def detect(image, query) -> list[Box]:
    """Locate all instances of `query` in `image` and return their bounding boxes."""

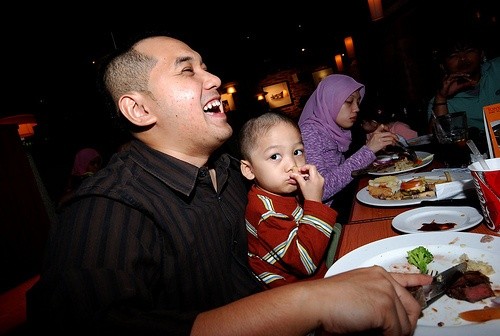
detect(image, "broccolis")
[408,247,434,273]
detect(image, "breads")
[369,176,400,200]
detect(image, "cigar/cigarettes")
[463,73,472,81]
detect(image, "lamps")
[367,0,384,21]
[335,53,344,71]
[344,34,356,60]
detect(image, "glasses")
[89,161,101,167]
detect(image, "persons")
[358,109,418,151]
[239,108,337,289]
[23,27,421,336]
[298,75,400,208]
[427,25,500,133]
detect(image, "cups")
[434,110,471,170]
[467,157,500,234]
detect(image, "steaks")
[445,272,492,303]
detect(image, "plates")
[367,151,434,176]
[405,129,456,145]
[355,172,471,206]
[323,231,500,336]
[391,205,484,233]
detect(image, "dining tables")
[314,159,500,336]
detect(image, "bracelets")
[433,102,447,106]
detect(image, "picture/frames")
[262,80,294,109]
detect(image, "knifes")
[408,262,467,309]
